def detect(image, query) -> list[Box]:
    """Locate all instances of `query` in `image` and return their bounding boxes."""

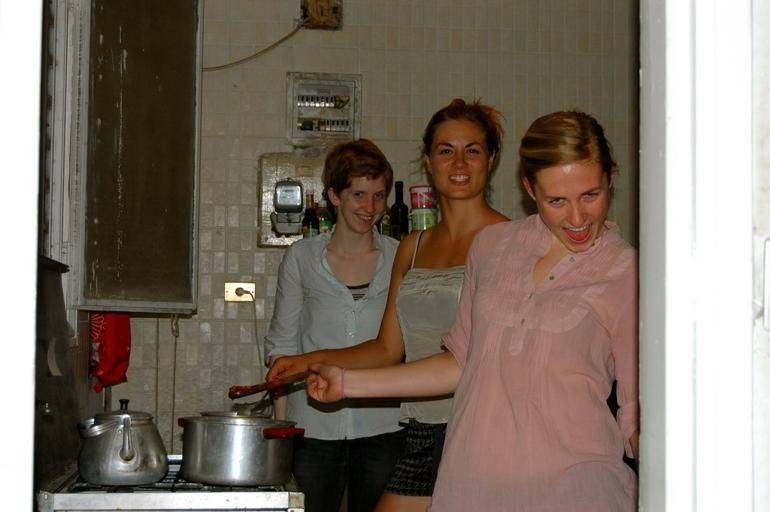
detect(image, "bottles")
[299,185,320,238]
[390,179,409,243]
[315,192,333,236]
[407,183,440,232]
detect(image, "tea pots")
[82,394,169,488]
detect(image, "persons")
[263,137,412,512]
[303,109,640,512]
[265,86,511,512]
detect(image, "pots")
[178,408,301,485]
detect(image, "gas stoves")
[51,449,305,509]
